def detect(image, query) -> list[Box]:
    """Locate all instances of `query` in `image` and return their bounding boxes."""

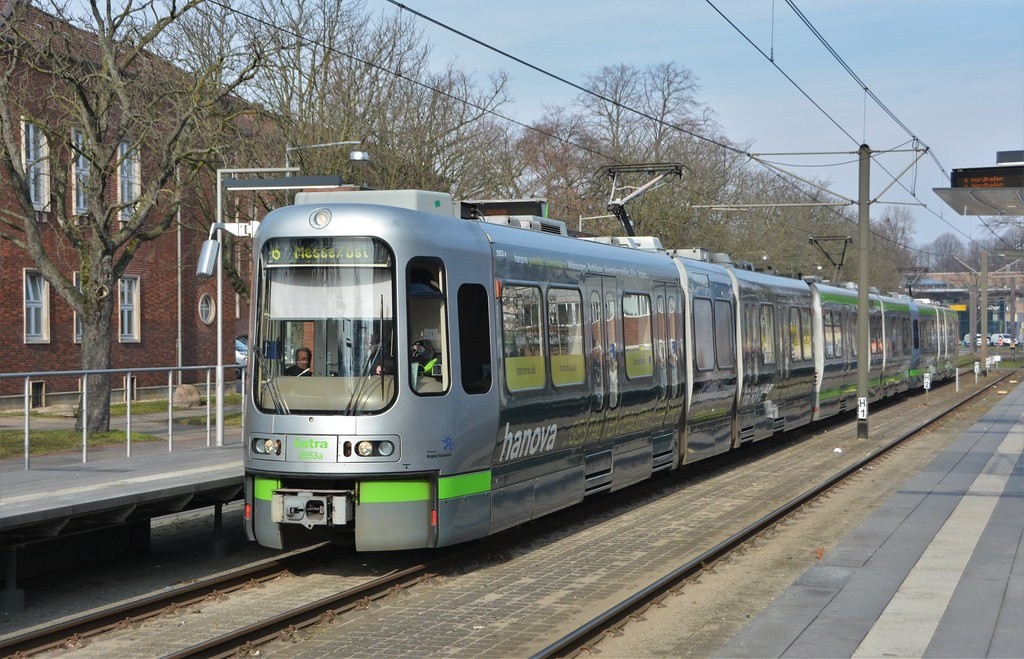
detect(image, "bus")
[194,161,962,553]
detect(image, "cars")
[234,335,249,380]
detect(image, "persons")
[341,321,394,374]
[284,347,312,377]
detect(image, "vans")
[963,333,991,348]
[990,333,1019,346]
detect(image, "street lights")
[285,139,369,207]
